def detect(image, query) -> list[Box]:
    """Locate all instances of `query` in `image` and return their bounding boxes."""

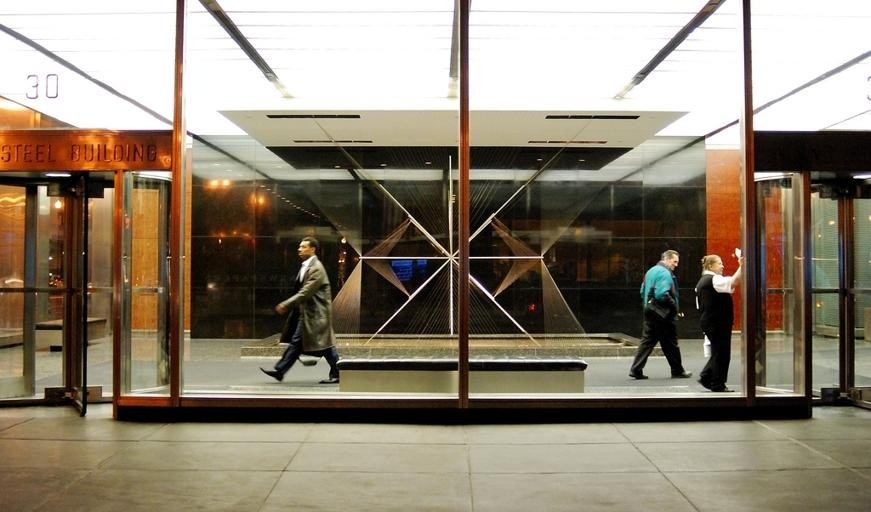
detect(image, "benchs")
[336,356,588,395]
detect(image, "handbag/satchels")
[644,297,674,328]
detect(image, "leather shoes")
[318,377,339,384]
[671,371,692,378]
[258,366,284,382]
[629,370,648,379]
[697,377,735,392]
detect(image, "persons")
[628,250,692,380]
[695,255,744,392]
[259,237,340,384]
